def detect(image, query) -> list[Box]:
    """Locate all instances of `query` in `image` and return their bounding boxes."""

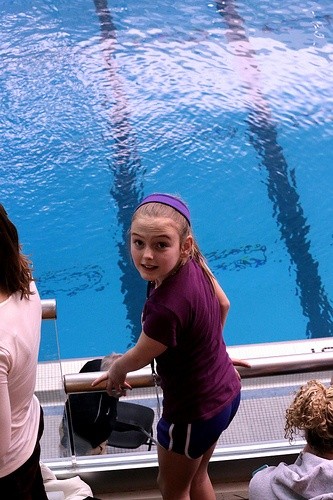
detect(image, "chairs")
[107,401,167,452]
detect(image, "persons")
[0,204,48,500]
[248,380,333,500]
[91,192,241,500]
